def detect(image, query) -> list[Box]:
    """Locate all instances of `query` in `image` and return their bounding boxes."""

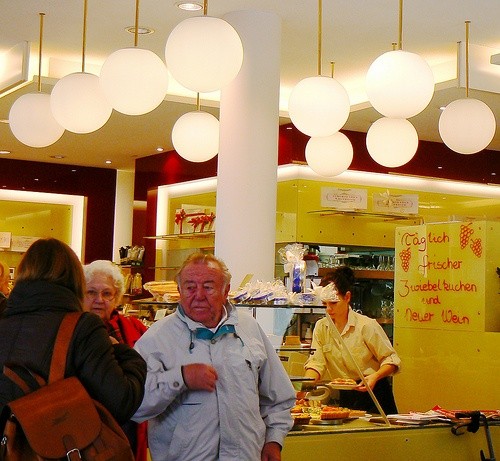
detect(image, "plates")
[326,383,360,392]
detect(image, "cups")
[274,297,287,305]
[302,293,316,303]
[286,336,300,346]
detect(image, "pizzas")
[290,406,311,413]
[331,378,357,385]
[320,405,351,420]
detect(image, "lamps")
[8,0,496,177]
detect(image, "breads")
[294,391,318,407]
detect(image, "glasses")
[83,287,117,302]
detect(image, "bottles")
[305,321,315,345]
[134,272,143,294]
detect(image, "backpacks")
[0,311,135,461]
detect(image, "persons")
[129,254,297,461]
[0,238,148,461]
[81,260,149,461]
[305,266,402,415]
[0,262,10,304]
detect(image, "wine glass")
[378,297,394,318]
[366,254,395,270]
